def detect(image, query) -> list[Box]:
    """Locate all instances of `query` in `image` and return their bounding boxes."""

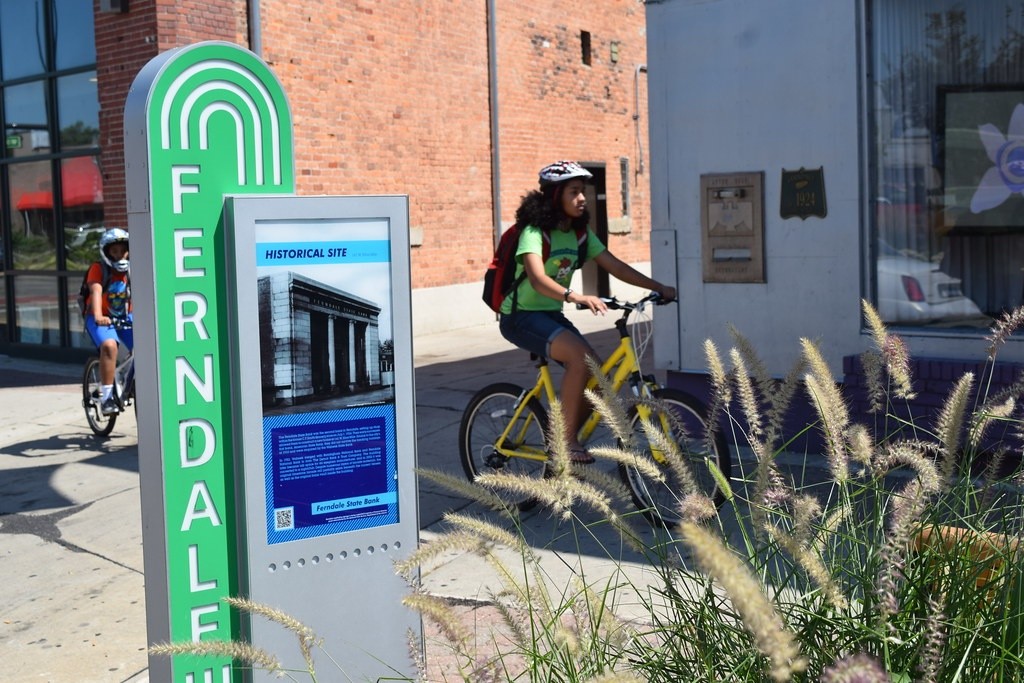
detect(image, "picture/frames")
[933,85,1024,235]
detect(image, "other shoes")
[101,397,120,414]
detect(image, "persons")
[497,160,677,463]
[78,228,134,414]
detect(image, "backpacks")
[484,219,589,314]
[77,261,131,319]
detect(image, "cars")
[62,214,105,250]
[872,182,980,328]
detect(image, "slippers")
[545,446,596,465]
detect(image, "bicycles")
[81,317,137,437]
[456,288,732,533]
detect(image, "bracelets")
[563,289,574,304]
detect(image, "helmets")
[99,228,129,272]
[539,161,594,190]
